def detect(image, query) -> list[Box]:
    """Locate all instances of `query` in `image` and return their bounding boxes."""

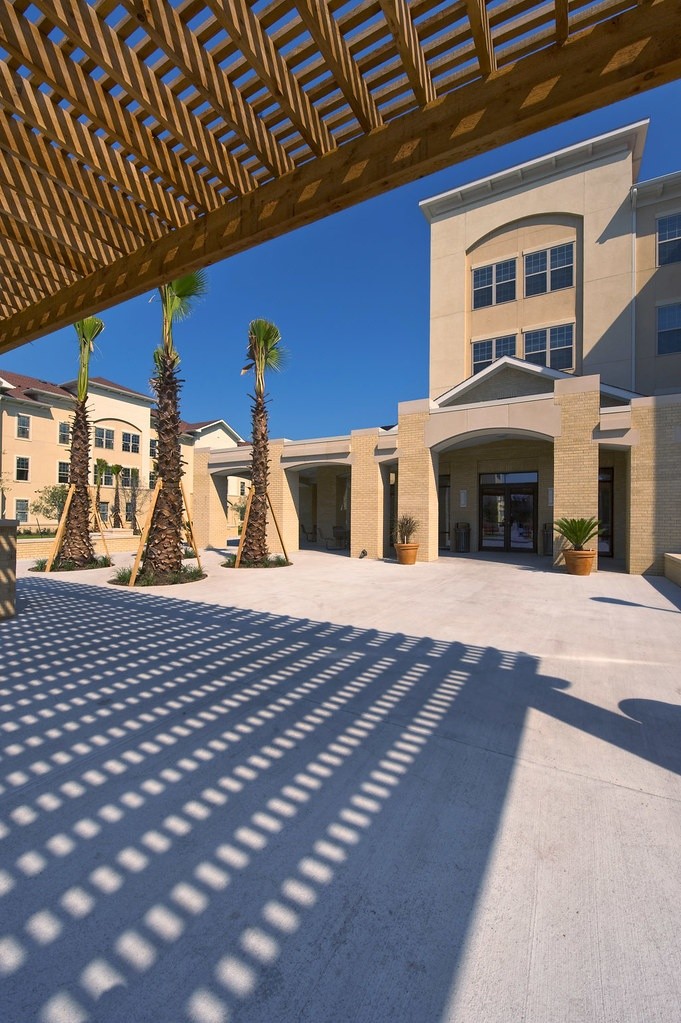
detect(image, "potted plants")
[390,515,422,566]
[550,515,610,575]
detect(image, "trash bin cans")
[453,521,471,553]
[543,523,554,556]
